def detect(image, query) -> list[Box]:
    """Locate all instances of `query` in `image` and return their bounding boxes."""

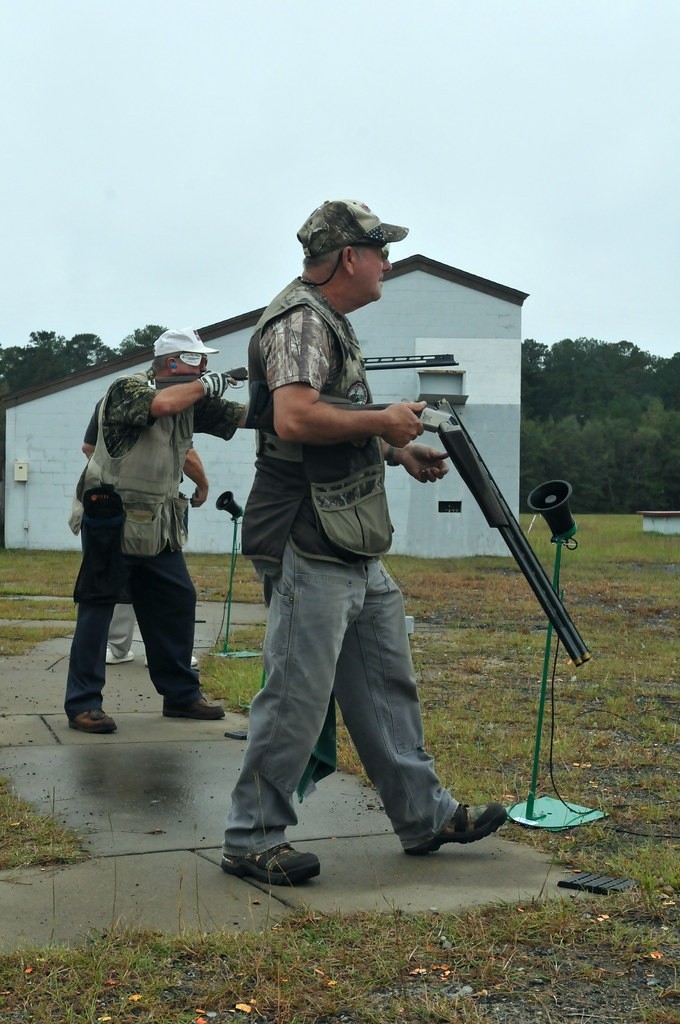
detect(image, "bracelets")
[387,446,400,466]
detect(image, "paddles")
[143,350,460,390]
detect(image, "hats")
[297,200,409,257]
[153,327,220,357]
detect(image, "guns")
[315,393,592,668]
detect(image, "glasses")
[174,352,207,367]
[349,242,390,261]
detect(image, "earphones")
[170,362,177,369]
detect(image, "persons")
[63,327,248,734]
[220,199,508,886]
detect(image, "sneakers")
[163,697,225,719]
[69,708,117,733]
[404,803,507,855]
[222,842,320,885]
